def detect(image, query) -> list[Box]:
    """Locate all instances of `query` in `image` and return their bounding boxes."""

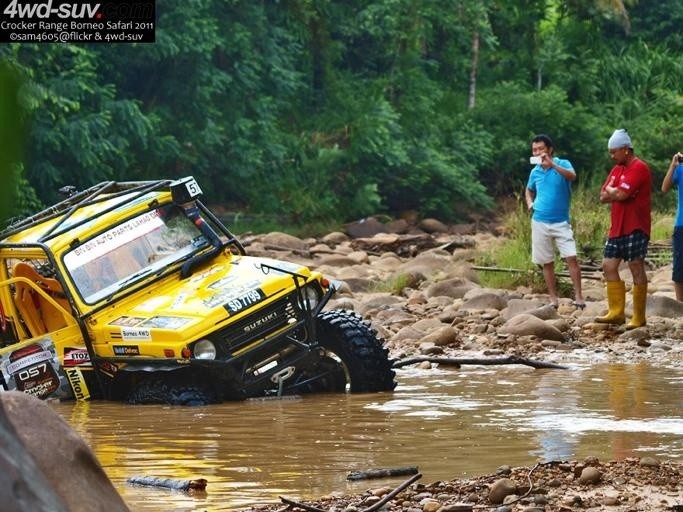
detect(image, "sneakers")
[570,300,586,308]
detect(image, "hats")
[608,129,633,150]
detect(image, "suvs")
[0,175,396,407]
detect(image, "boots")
[594,280,626,323]
[625,283,647,330]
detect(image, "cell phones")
[529,158,543,165]
[678,157,683,163]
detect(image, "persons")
[535,364,658,462]
[662,152,683,303]
[525,135,586,307]
[595,129,655,329]
